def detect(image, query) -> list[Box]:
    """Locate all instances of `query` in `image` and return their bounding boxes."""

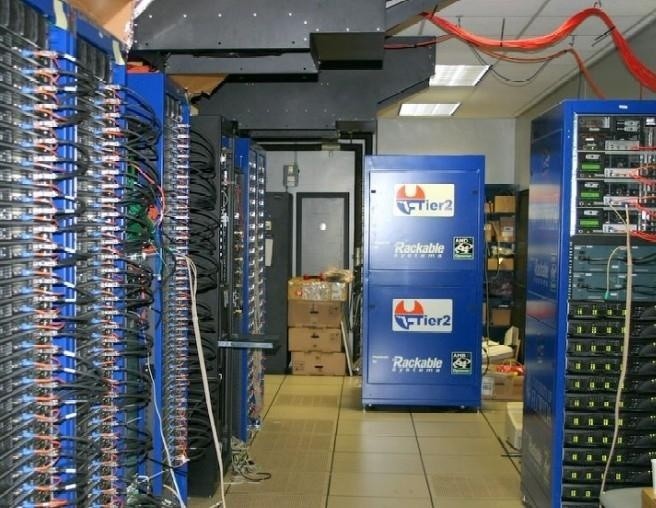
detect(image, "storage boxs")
[287,277,349,377]
[482,196,525,401]
